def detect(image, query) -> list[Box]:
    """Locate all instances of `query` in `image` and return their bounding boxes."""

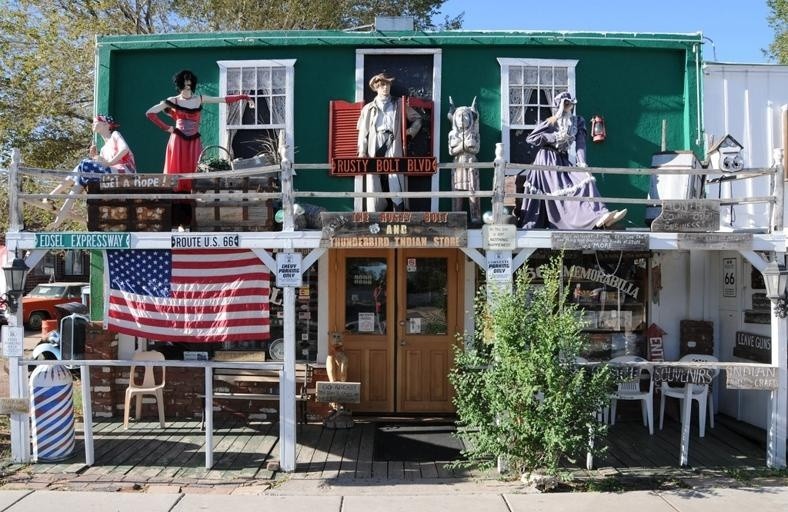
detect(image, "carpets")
[371,426,468,461]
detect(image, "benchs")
[199,364,313,427]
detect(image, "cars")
[1,281,89,333]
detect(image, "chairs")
[123,351,166,428]
[535,353,720,468]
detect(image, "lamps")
[590,115,607,143]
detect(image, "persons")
[446,106,482,223]
[518,91,628,230]
[40,113,136,228]
[354,72,422,212]
[144,70,251,207]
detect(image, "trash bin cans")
[29,364,75,463]
[55,302,88,333]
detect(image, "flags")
[101,249,271,343]
